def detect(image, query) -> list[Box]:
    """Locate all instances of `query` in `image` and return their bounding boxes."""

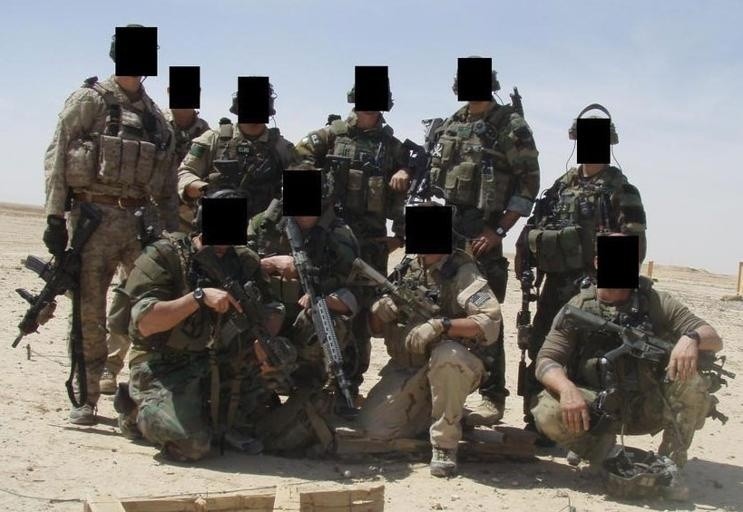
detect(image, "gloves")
[404,321,441,356]
[43,215,68,260]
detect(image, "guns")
[517,232,543,432]
[282,216,357,420]
[346,258,494,367]
[11,203,102,349]
[193,277,298,391]
[556,304,737,378]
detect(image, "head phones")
[453,70,500,96]
[569,104,619,145]
[280,169,333,199]
[230,90,276,116]
[347,85,394,110]
[109,24,145,63]
[193,188,240,232]
[403,184,456,215]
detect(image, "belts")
[73,192,147,210]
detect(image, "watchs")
[193,286,206,308]
[441,316,451,335]
[491,223,507,238]
[683,329,700,345]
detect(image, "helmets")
[600,456,659,497]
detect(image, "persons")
[41,27,178,423]
[404,54,540,420]
[513,115,648,447]
[293,71,427,412]
[175,80,301,222]
[354,200,502,479]
[114,188,286,460]
[161,80,213,233]
[527,233,724,502]
[246,162,361,414]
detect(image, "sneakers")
[429,450,460,478]
[465,394,504,426]
[71,399,95,425]
[225,428,261,453]
[99,366,117,393]
[118,407,141,438]
